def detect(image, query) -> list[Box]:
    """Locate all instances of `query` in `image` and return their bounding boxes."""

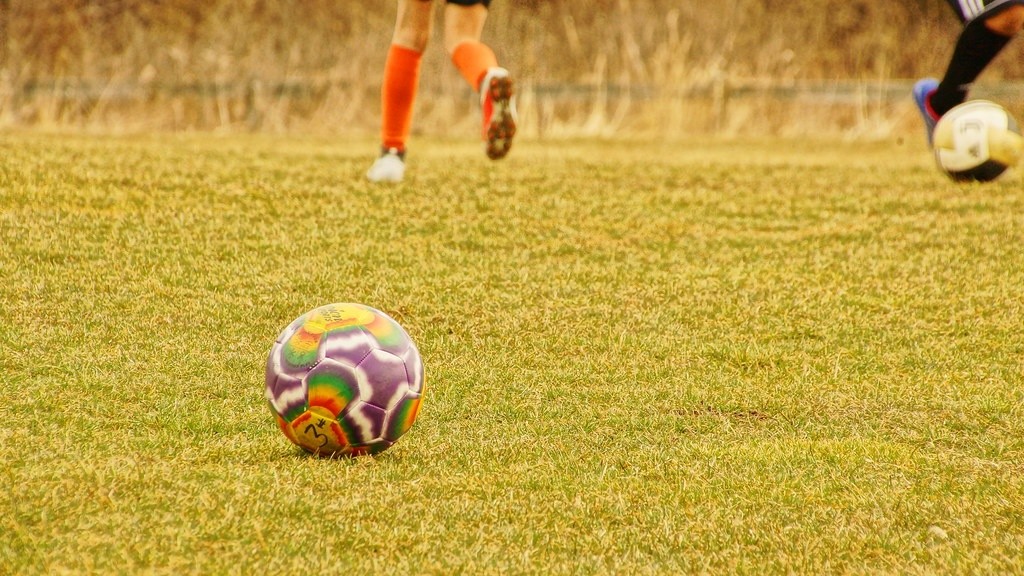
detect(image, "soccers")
[932,99,1023,183]
[264,302,427,457]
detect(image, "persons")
[914,0,1024,149]
[365,1,517,185]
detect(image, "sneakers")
[367,147,405,183]
[913,78,941,157]
[479,67,518,160]
